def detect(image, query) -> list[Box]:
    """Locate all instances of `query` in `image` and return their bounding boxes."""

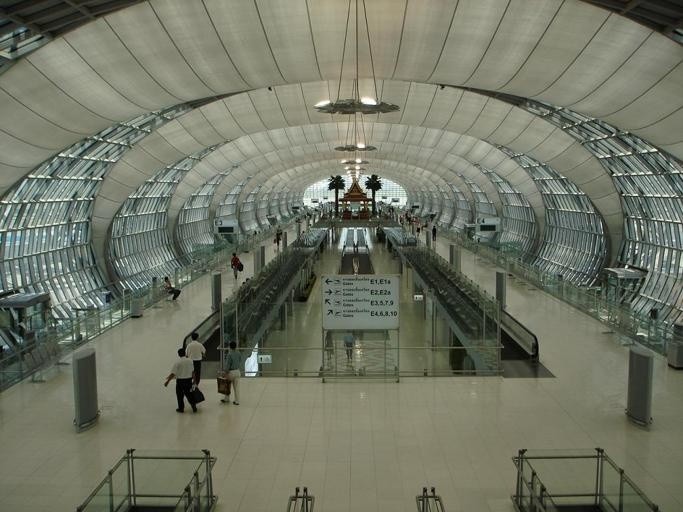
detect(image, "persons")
[162,348,197,412]
[274,229,281,246]
[277,225,283,237]
[230,252,239,280]
[163,277,180,301]
[218,340,241,405]
[380,201,428,237]
[431,225,436,241]
[183,332,206,388]
[323,331,334,366]
[342,332,355,359]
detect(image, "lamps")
[313,1,399,153]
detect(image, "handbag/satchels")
[189,381,205,404]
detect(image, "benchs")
[23,337,70,383]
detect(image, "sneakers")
[176,408,185,413]
[192,407,198,413]
[220,399,231,404]
[233,401,240,405]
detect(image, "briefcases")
[216,374,231,395]
